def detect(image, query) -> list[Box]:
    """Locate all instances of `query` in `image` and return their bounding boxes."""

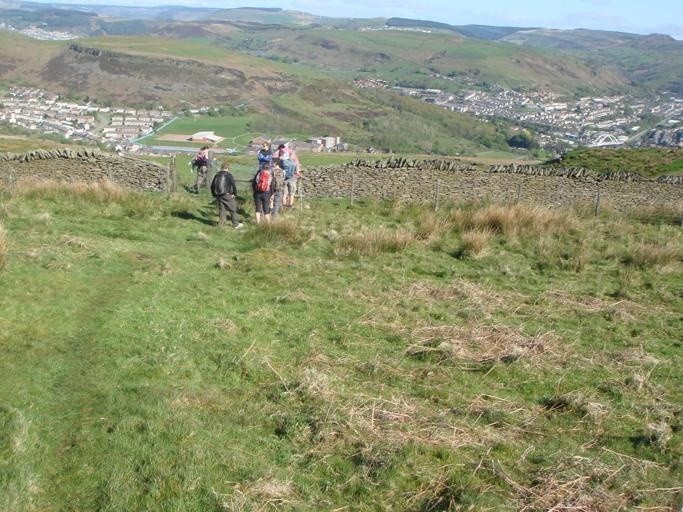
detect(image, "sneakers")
[233,223,243,229]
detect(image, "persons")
[251,142,301,226]
[194,145,211,194]
[210,161,243,229]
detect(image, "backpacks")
[272,169,284,192]
[255,169,270,193]
[214,172,229,196]
[282,159,296,176]
